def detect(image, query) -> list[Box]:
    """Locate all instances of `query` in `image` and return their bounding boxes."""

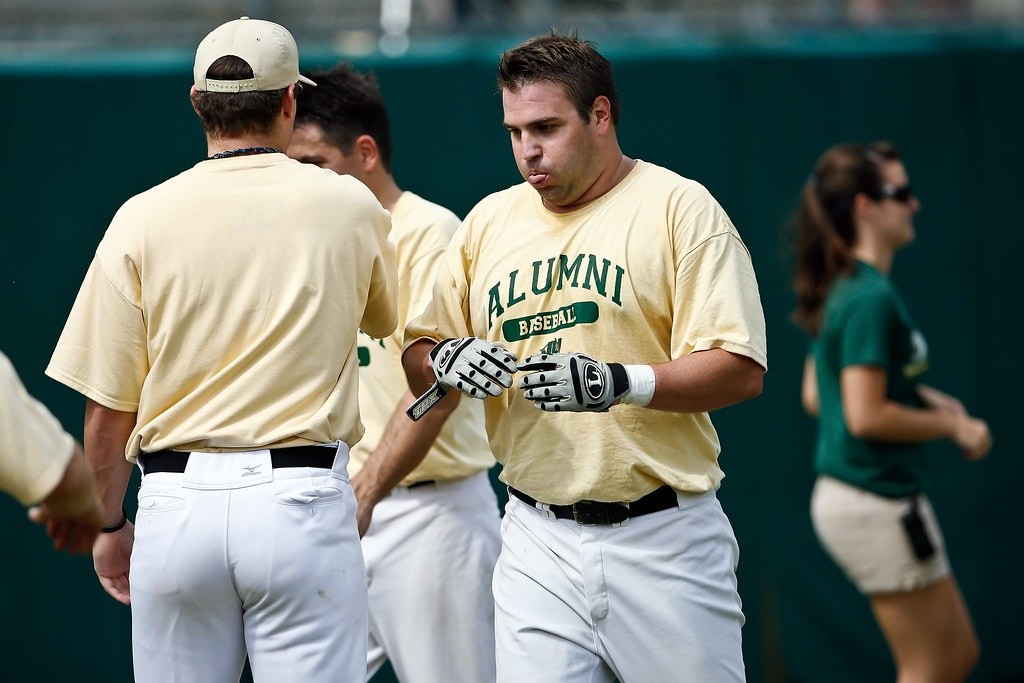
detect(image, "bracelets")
[101,507,128,533]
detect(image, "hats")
[192,17,318,94]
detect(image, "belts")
[405,480,437,491]
[135,445,338,475]
[508,484,678,527]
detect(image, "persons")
[400,33,766,683]
[801,140,989,683]
[0,13,501,683]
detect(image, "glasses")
[877,184,913,204]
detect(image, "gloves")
[403,337,519,422]
[515,351,633,414]
